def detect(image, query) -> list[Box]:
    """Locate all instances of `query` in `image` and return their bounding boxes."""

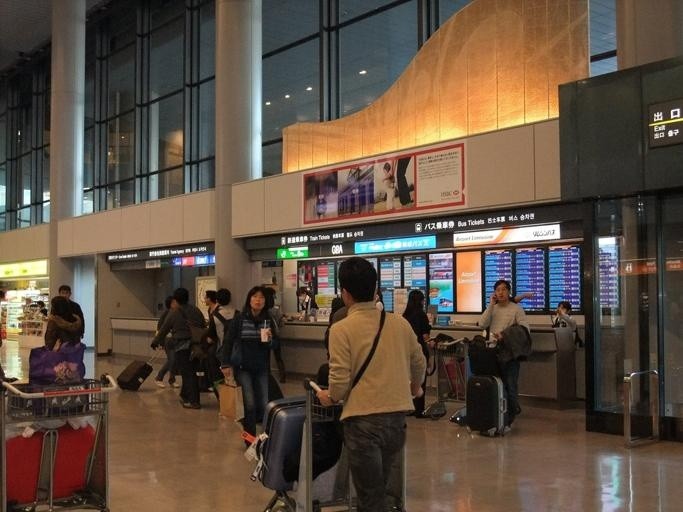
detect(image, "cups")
[259,328,270,343]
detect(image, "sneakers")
[179,399,201,409]
[155,380,166,388]
[170,382,180,387]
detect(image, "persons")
[202,288,219,341]
[401,289,436,420]
[34,300,48,317]
[43,294,81,351]
[374,289,383,302]
[315,257,427,512]
[381,157,413,209]
[265,286,282,329]
[204,288,240,424]
[508,291,535,303]
[220,284,288,449]
[23,296,33,308]
[295,286,319,321]
[380,162,395,212]
[58,285,85,340]
[477,279,532,436]
[154,295,180,388]
[375,299,386,311]
[150,286,208,409]
[551,300,578,331]
[324,296,344,363]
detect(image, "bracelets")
[327,394,339,404]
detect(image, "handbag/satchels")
[422,402,447,421]
[215,340,243,366]
[217,370,245,421]
[434,333,456,354]
[29,344,87,416]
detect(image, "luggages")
[117,348,162,393]
[256,395,344,491]
[466,375,507,437]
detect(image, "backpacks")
[214,309,241,336]
[468,335,500,377]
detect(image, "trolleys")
[256,378,407,512]
[0,370,118,512]
[421,335,471,421]
